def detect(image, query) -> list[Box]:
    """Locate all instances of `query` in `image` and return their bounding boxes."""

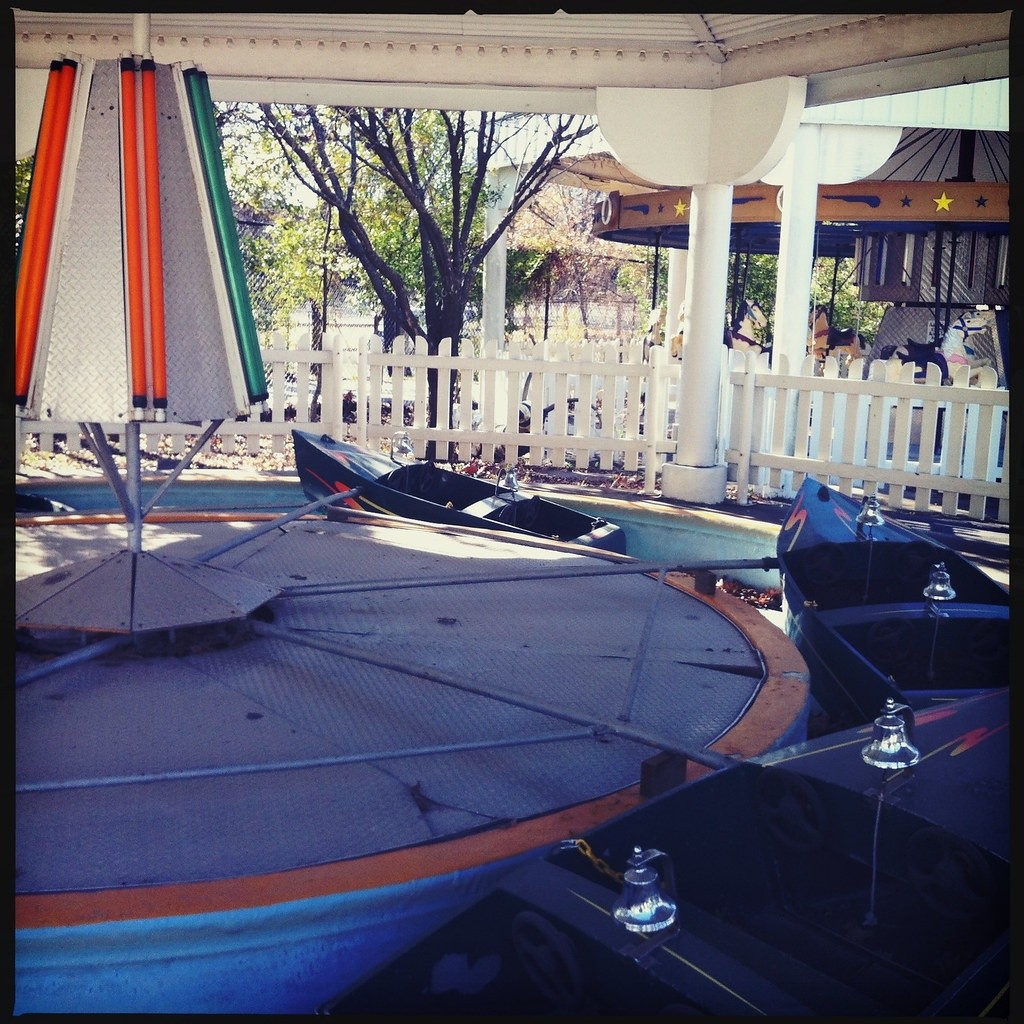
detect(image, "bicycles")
[501,395,626,474]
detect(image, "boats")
[285,419,625,564]
[777,466,1011,717]
[321,692,1024,1024]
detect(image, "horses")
[644,296,996,386]
